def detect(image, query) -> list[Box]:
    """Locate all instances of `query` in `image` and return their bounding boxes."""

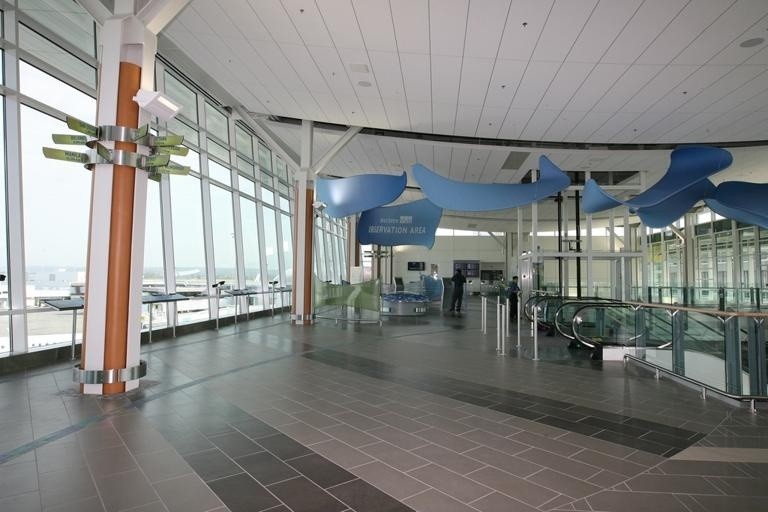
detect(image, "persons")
[507,276,520,323]
[449,268,466,312]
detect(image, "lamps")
[132,87,182,123]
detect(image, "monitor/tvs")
[407,262,425,271]
